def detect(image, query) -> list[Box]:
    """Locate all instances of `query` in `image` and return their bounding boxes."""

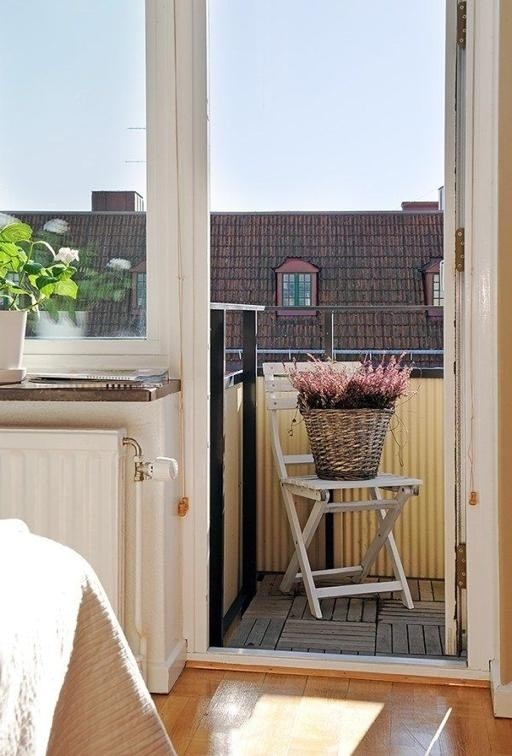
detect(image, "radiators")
[0,425,127,634]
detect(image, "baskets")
[296,393,396,482]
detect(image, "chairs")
[263,362,424,619]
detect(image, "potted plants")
[0,221,80,383]
[285,353,423,481]
[31,219,129,337]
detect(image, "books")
[27,367,170,384]
